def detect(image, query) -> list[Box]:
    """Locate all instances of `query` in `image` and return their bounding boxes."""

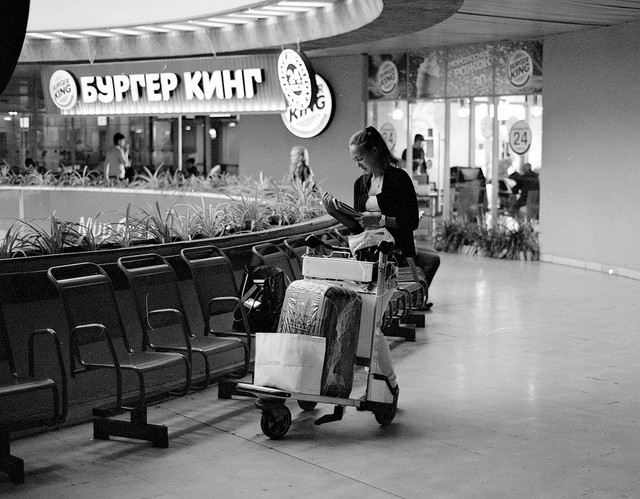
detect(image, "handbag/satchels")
[347,228,395,259]
[355,241,394,284]
[254,333,326,395]
[232,264,287,331]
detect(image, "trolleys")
[219,234,402,438]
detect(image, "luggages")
[277,279,362,398]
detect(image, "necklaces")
[373,176,381,188]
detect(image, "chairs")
[283,236,305,279]
[311,225,428,342]
[252,243,297,281]
[117,252,250,391]
[180,244,256,398]
[48,262,192,448]
[1,309,68,484]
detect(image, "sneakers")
[356,384,399,411]
[425,303,433,309]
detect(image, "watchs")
[379,215,386,228]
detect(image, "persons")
[401,134,428,174]
[207,164,226,180]
[321,126,421,405]
[513,163,540,210]
[185,158,197,177]
[288,145,315,192]
[104,132,130,180]
